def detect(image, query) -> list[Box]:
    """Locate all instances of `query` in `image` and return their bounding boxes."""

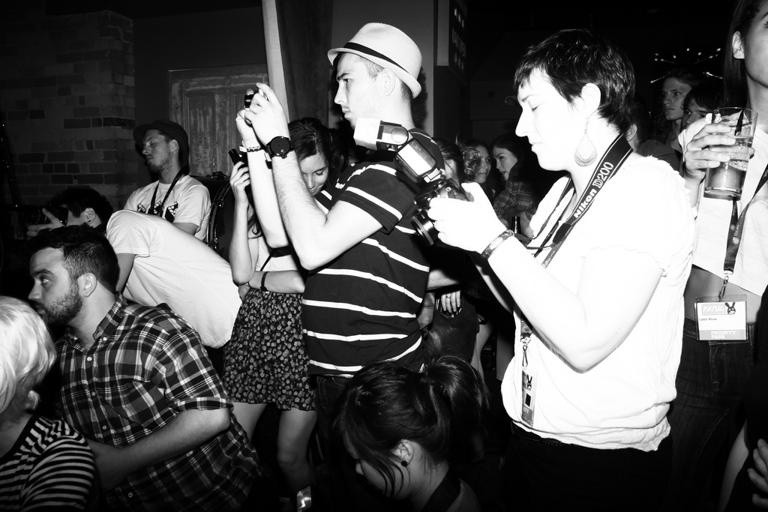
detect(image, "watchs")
[267,136,295,158]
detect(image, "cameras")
[240,88,261,112]
[2,199,66,242]
[276,479,314,511]
[376,119,472,248]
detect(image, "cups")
[703,105,758,201]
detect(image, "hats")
[133,120,188,151]
[327,22,422,99]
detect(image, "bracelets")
[241,141,263,152]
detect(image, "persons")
[415,0,767,511]
[224,117,334,511]
[27,228,285,511]
[235,21,446,512]
[332,355,490,512]
[0,295,96,512]
[26,188,246,380]
[122,121,211,243]
